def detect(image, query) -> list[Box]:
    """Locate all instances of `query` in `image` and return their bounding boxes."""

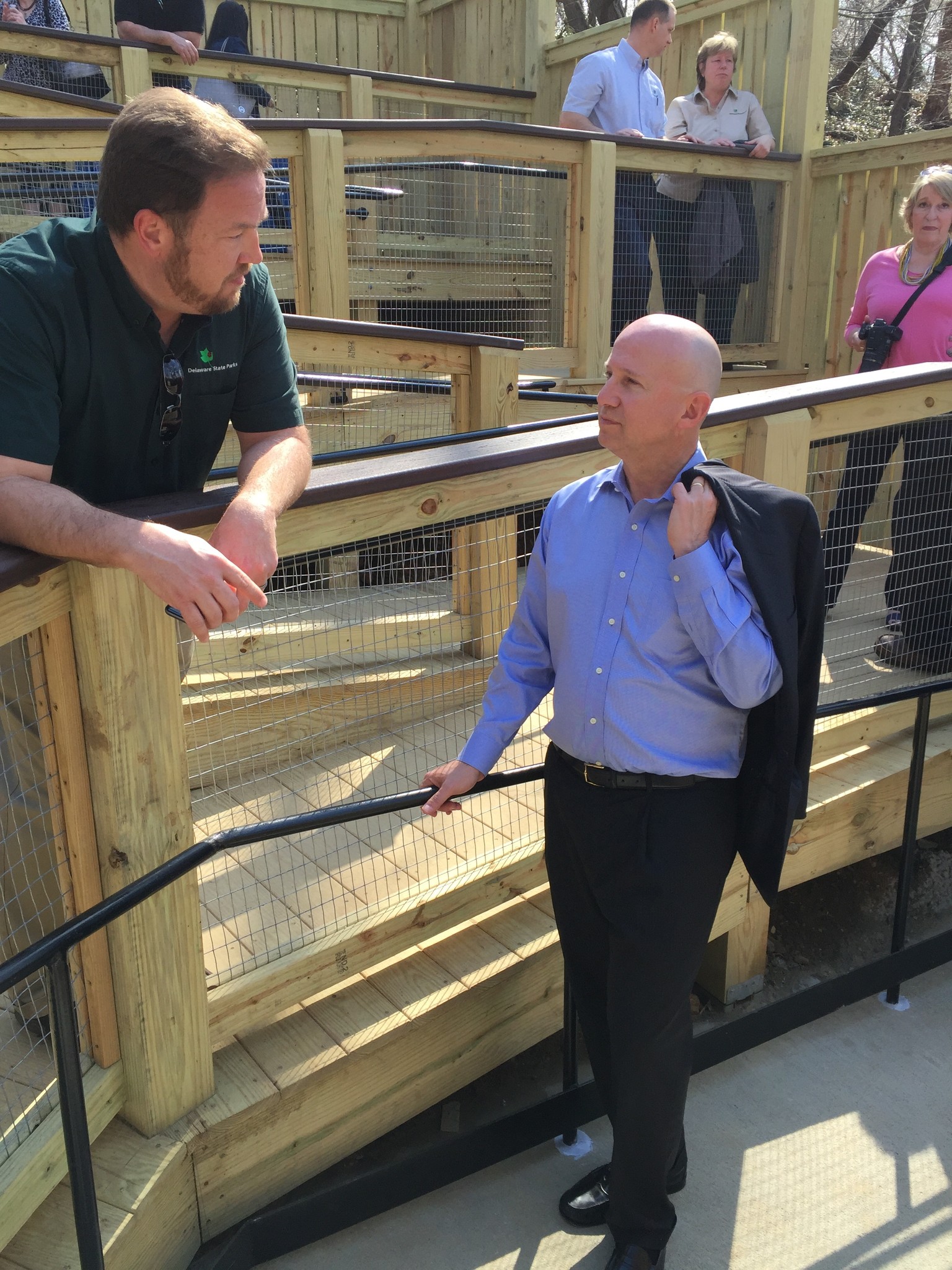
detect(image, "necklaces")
[17,0,36,11]
[899,233,952,286]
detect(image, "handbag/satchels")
[39,0,111,100]
[196,38,256,118]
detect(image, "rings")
[692,482,707,492]
[13,16,15,20]
[859,346,862,351]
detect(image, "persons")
[558,0,707,346]
[874,425,952,676]
[656,31,776,371]
[421,314,785,1270]
[0,86,315,1040]
[204,1,276,117]
[114,0,207,98]
[822,168,952,630]
[0,0,72,217]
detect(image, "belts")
[553,745,705,788]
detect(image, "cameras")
[858,318,903,373]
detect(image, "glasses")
[240,4,245,11]
[158,349,185,445]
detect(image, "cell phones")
[165,591,215,625]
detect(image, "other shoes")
[875,635,952,671]
[722,363,734,371]
[12,1010,52,1041]
[886,612,902,631]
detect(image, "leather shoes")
[605,1242,666,1270]
[558,1161,687,1227]
